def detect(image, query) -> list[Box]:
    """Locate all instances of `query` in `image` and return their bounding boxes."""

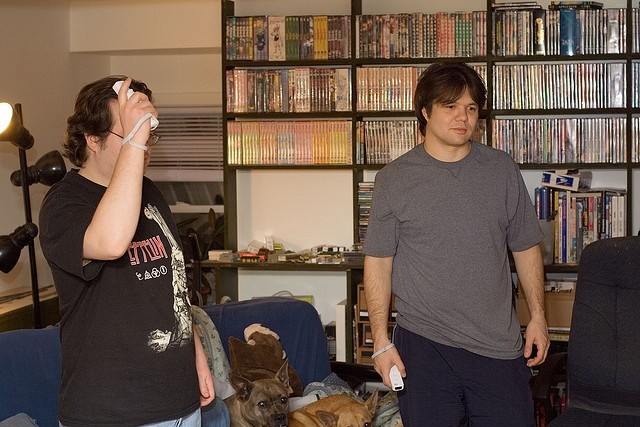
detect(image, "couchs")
[0,297,331,427]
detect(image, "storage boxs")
[513,278,578,328]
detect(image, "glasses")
[106,131,161,149]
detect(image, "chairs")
[545,237,639,427]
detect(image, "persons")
[38,74,215,426]
[363,59,550,426]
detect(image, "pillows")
[322,371,354,392]
[303,382,364,405]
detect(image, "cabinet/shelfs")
[218,0,638,346]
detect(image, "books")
[492,114,627,164]
[354,11,487,59]
[489,1,627,56]
[358,181,375,243]
[356,62,426,111]
[226,15,351,60]
[631,117,639,163]
[491,59,627,109]
[468,118,487,146]
[356,116,425,164]
[534,170,627,265]
[468,63,487,110]
[226,118,353,166]
[632,60,639,109]
[632,7,640,54]
[226,66,352,112]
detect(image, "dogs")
[288,386,379,427]
[222,358,294,427]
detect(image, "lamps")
[0,101,67,324]
